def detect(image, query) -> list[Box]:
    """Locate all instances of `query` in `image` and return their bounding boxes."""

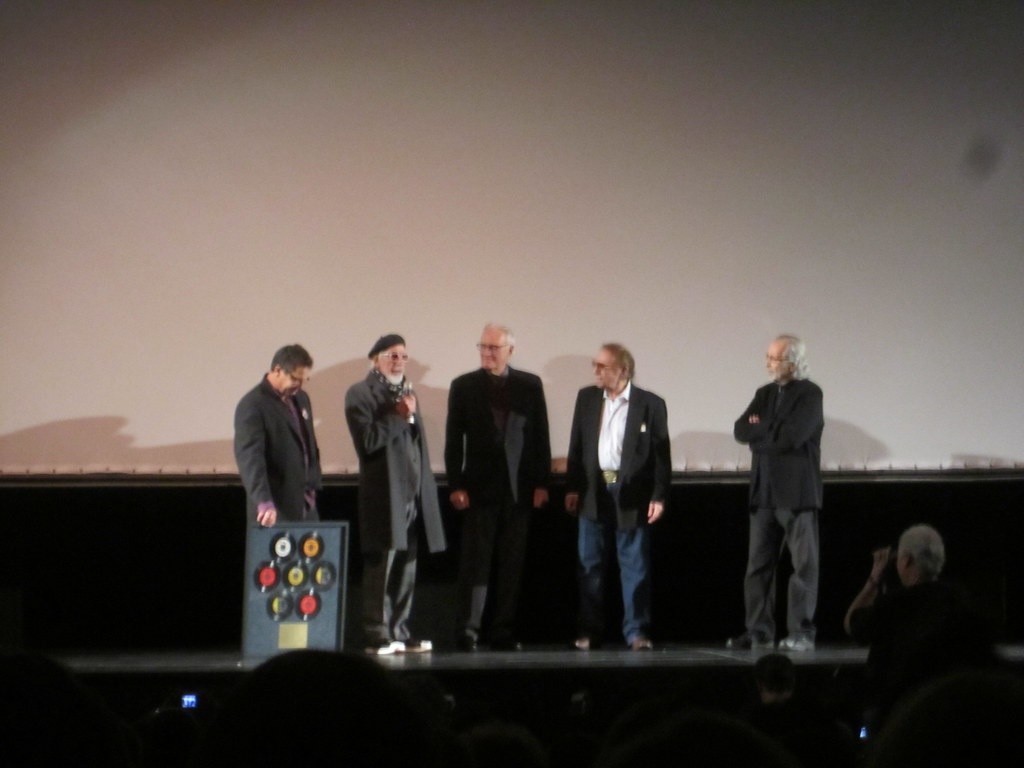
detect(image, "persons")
[443,322,552,653]
[0,661,1024,768]
[844,525,991,733]
[565,345,672,651]
[727,335,824,652]
[343,335,445,654]
[234,344,321,528]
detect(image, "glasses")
[376,353,409,362]
[476,343,510,352]
[281,366,309,384]
[592,359,624,370]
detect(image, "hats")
[368,335,405,358]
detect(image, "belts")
[599,470,620,483]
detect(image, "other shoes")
[457,640,478,652]
[491,638,522,652]
[625,635,652,651]
[367,642,409,655]
[725,633,774,651]
[406,637,432,655]
[569,634,601,651]
[779,632,814,653]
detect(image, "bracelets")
[869,576,881,589]
